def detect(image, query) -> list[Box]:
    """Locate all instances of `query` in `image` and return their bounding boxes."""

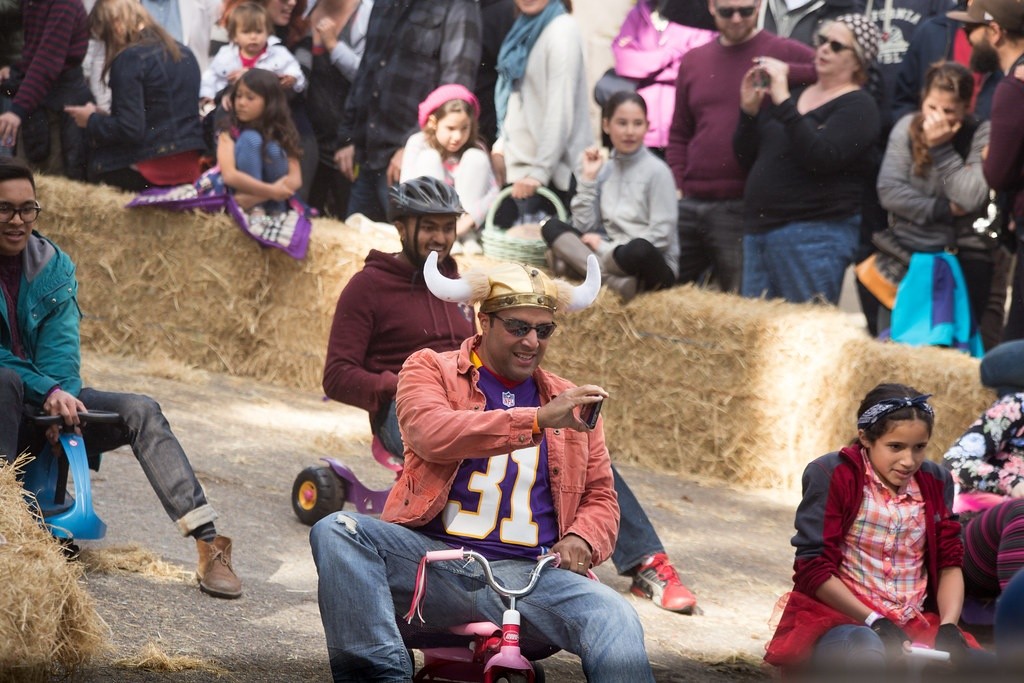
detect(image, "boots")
[545,242,626,279]
[551,231,636,304]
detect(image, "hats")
[945,0,1024,34]
[418,84,479,127]
[838,14,879,63]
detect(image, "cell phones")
[579,394,604,430]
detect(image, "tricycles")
[403,549,559,683]
[291,432,408,525]
[13,406,123,561]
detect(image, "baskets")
[479,186,568,266]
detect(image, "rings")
[577,562,584,566]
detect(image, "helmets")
[422,251,603,314]
[387,176,466,219]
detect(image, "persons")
[759,380,1001,682]
[0,1,1024,355]
[306,245,667,683]
[323,174,699,616]
[0,161,247,600]
[939,390,1024,615]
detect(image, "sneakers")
[629,551,695,613]
[194,536,242,598]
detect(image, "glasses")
[960,22,986,38]
[0,201,41,224]
[488,312,558,340]
[818,34,853,54]
[714,2,756,17]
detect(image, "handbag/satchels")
[856,252,908,310]
[593,72,640,107]
